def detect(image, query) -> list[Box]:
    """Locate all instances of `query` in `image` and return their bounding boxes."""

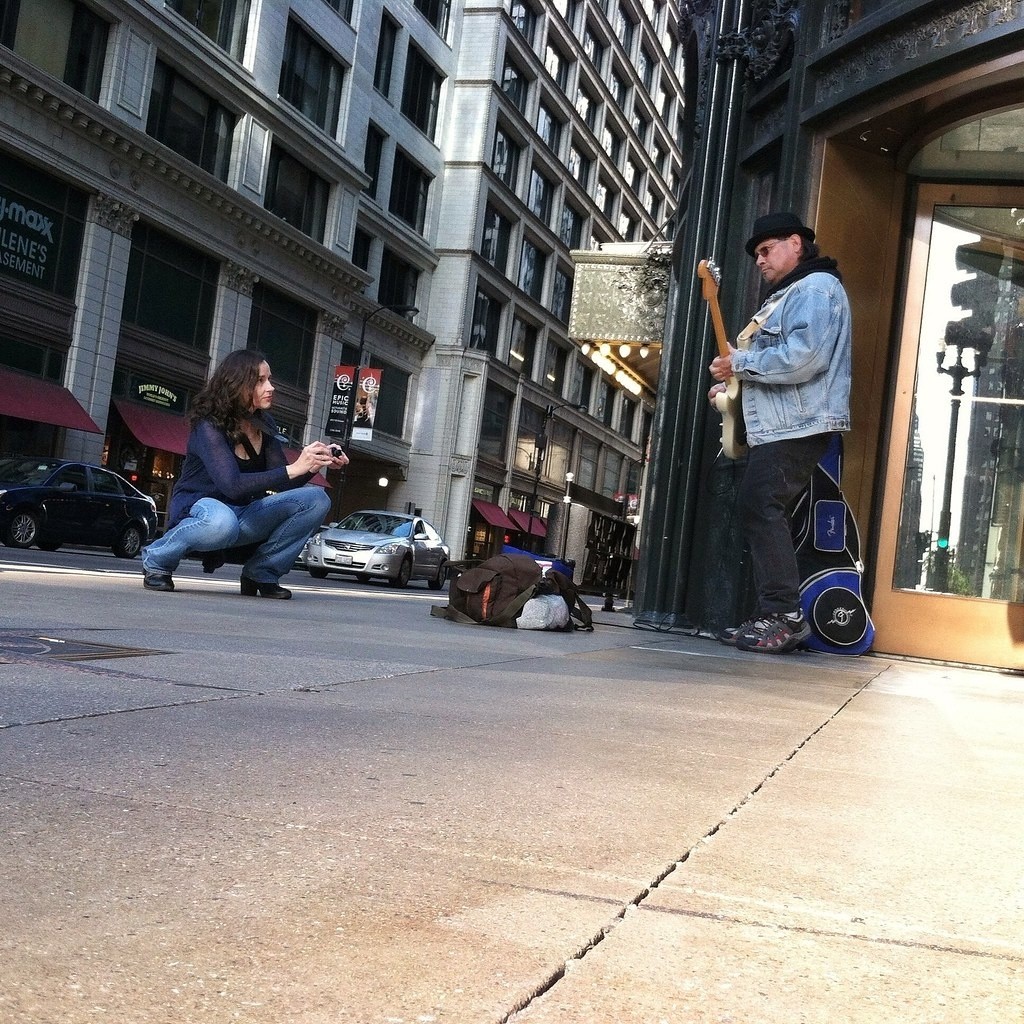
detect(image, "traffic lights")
[945,240,1004,347]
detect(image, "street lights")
[334,302,419,528]
[527,402,587,551]
[928,337,985,592]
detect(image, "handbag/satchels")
[431,552,592,634]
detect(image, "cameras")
[324,445,344,458]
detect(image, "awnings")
[282,447,332,489]
[114,398,192,457]
[0,369,104,436]
[509,508,548,538]
[471,498,521,532]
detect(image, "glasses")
[754,236,790,262]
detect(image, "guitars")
[696,255,747,459]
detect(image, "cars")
[294,509,451,590]
[0,455,159,560]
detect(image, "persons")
[142,350,348,601]
[708,212,853,654]
[354,390,379,429]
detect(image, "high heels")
[240,575,291,599]
[142,567,174,591]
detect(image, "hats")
[745,212,815,256]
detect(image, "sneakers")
[719,615,766,646]
[736,612,811,653]
